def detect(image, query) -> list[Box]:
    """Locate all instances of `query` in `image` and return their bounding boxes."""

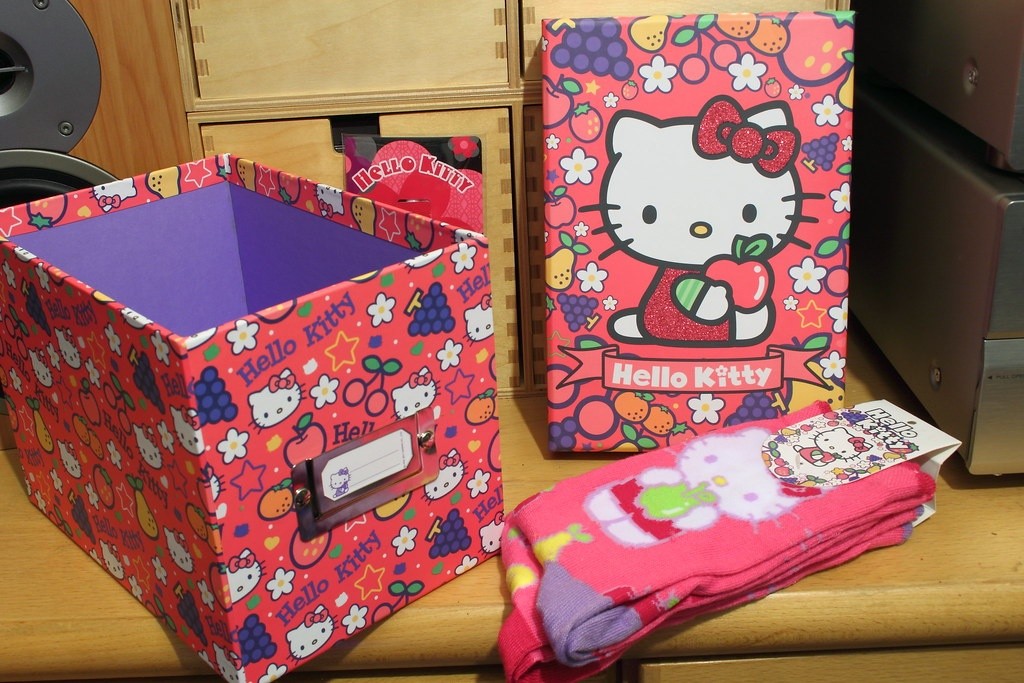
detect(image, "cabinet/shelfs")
[171,0,850,397]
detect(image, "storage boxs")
[0,153,504,683]
[541,11,854,449]
